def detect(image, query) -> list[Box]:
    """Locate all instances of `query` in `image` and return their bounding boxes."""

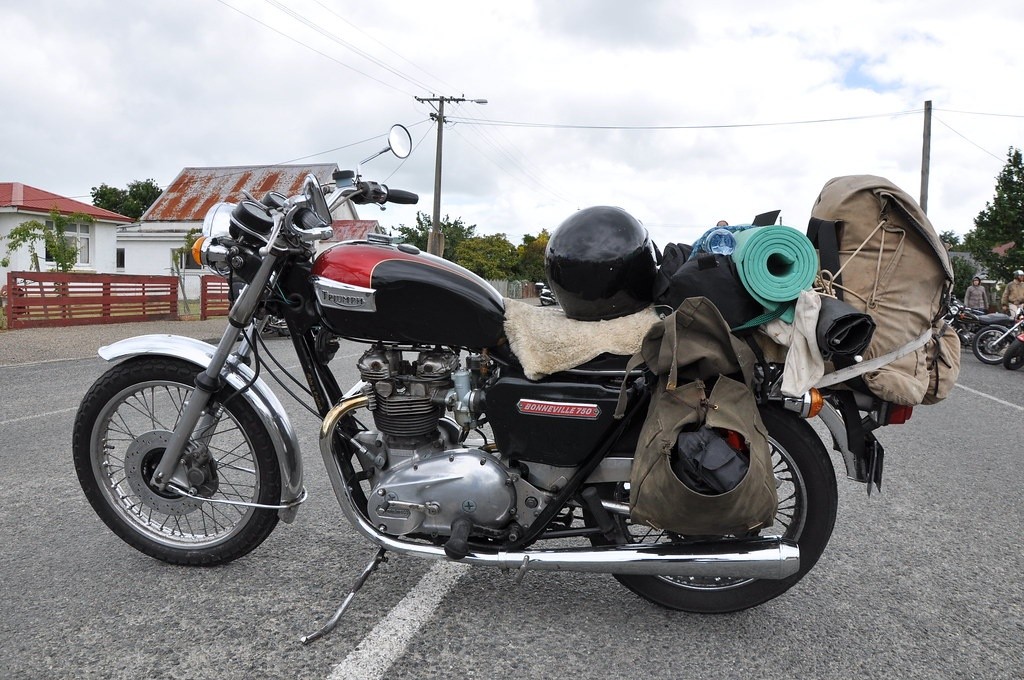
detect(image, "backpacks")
[806,175,961,407]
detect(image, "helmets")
[544,206,659,322]
[1013,270,1024,279]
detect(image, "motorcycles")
[1002,309,1024,369]
[534,279,558,308]
[972,304,1024,364]
[941,294,1016,354]
[71,122,914,619]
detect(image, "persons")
[716,220,729,227]
[964,276,989,311]
[1001,270,1024,319]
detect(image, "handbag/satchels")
[654,243,765,337]
[614,295,777,535]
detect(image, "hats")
[974,278,979,281]
